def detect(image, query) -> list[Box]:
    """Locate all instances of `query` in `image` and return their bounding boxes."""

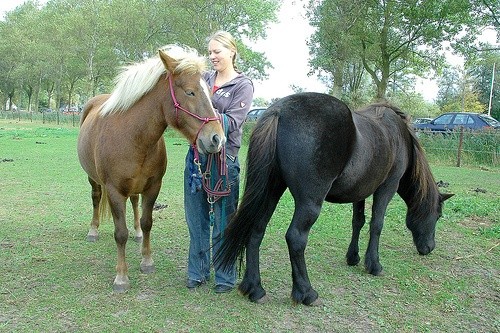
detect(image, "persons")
[183,30,254,292]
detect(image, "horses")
[77,43,227,294]
[196,92,455,307]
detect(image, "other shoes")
[187,281,202,288]
[215,285,231,293]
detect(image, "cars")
[244,107,267,122]
[410,118,433,130]
[412,112,496,138]
